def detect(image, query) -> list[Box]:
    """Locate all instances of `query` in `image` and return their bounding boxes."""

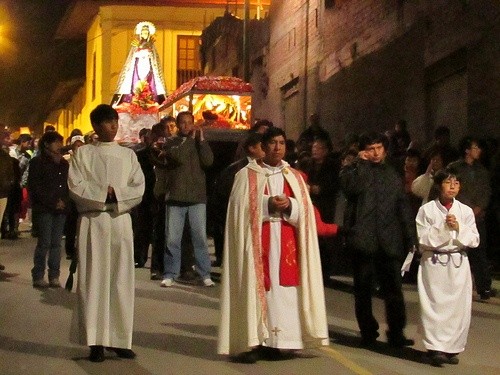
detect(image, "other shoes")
[265,347,280,360]
[89,347,104,362]
[10,231,21,238]
[360,332,379,346]
[182,271,195,280]
[33,279,50,288]
[428,350,446,364]
[151,272,160,280]
[447,353,459,363]
[0,264,5,270]
[50,277,61,288]
[480,290,490,302]
[388,337,415,351]
[135,262,144,268]
[66,254,75,259]
[114,348,134,359]
[238,351,256,363]
[2,232,8,239]
[490,288,498,296]
[212,260,221,267]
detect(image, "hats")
[71,135,85,145]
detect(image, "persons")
[340,120,498,298]
[160,112,215,288]
[138,111,337,286]
[107,21,166,104]
[400,171,480,364]
[68,104,144,360]
[161,115,178,140]
[337,136,416,349]
[216,126,331,360]
[0,125,89,292]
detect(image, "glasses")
[441,180,460,187]
[471,146,480,149]
[431,158,443,164]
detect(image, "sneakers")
[160,278,175,287]
[203,278,215,288]
